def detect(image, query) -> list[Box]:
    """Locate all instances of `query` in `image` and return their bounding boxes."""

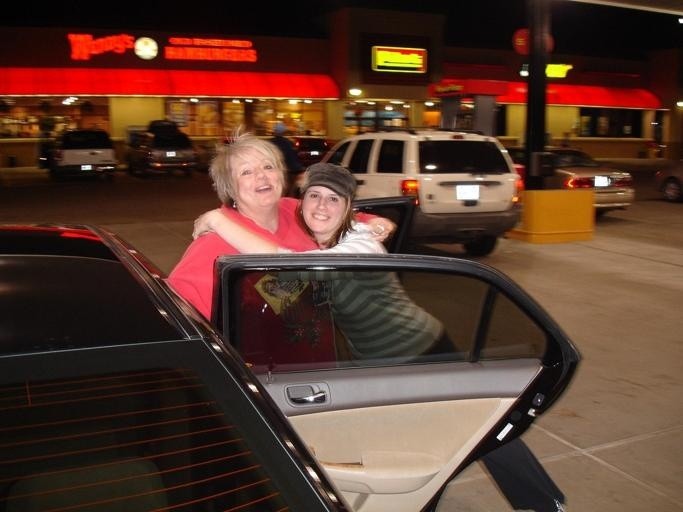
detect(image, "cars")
[499,142,639,218]
[650,158,682,205]
[278,131,329,168]
[2,219,584,511]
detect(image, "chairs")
[2,458,172,509]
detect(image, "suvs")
[288,123,526,261]
[35,120,119,187]
[123,117,201,177]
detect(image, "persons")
[183,162,565,511]
[162,125,338,366]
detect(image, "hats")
[301,164,357,203]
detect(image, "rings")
[376,224,385,231]
